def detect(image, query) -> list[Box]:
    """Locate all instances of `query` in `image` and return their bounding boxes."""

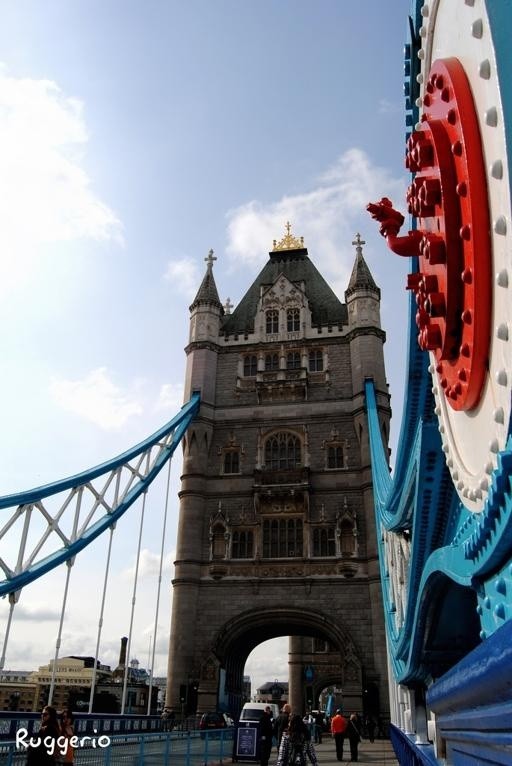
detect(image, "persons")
[53,707,76,766]
[24,705,59,766]
[166,710,176,732]
[159,710,168,732]
[254,703,377,766]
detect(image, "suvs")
[198,711,229,741]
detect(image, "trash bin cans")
[232,722,273,766]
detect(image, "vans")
[239,701,279,729]
